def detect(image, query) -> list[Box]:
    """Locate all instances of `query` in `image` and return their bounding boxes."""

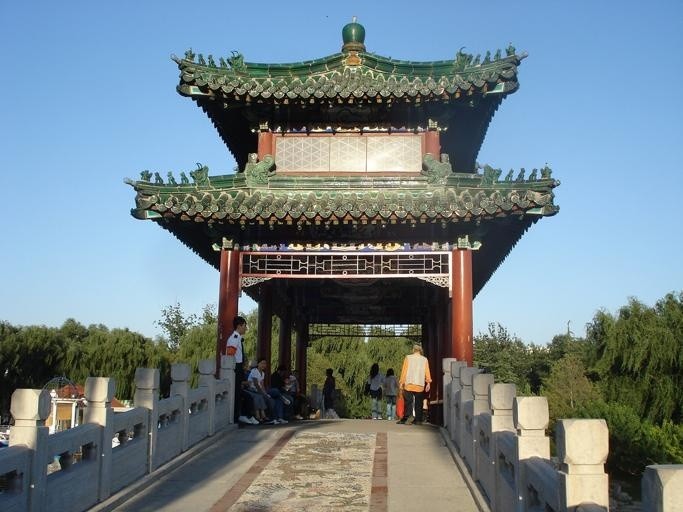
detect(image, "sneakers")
[373,416,395,421]
[295,414,303,419]
[396,419,406,423]
[239,416,258,424]
[411,419,422,425]
[260,417,289,425]
[279,395,291,406]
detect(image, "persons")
[383,368,400,420]
[363,363,383,419]
[396,345,432,424]
[225,317,260,424]
[322,368,335,410]
[240,357,303,425]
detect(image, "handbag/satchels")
[363,381,371,396]
[396,397,405,419]
